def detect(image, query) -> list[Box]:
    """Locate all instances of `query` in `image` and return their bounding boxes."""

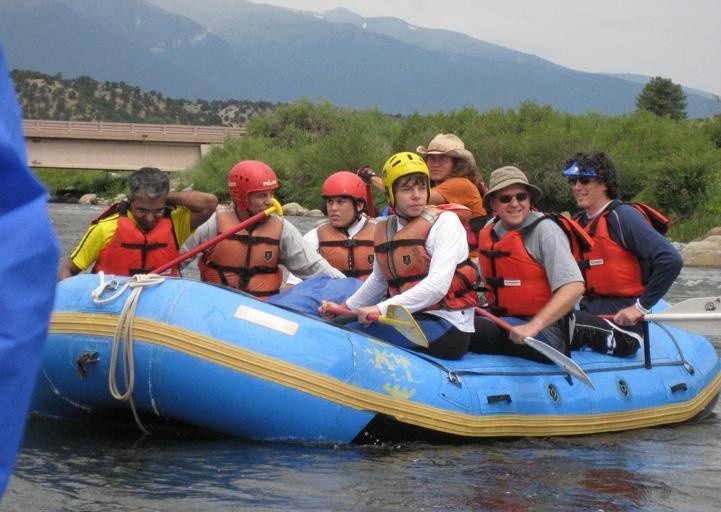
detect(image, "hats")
[482,165,544,212]
[416,133,479,177]
[561,152,605,179]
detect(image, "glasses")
[491,191,533,203]
[568,176,602,185]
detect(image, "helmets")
[229,160,281,211]
[321,171,369,207]
[382,151,431,208]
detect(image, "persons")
[357,134,489,278]
[285,171,376,292]
[562,151,684,358]
[57,166,217,280]
[180,160,347,302]
[468,166,586,365]
[318,151,489,360]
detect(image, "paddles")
[327,304,429,348]
[599,296,720,338]
[476,303,596,388]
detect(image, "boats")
[29,271,721,448]
